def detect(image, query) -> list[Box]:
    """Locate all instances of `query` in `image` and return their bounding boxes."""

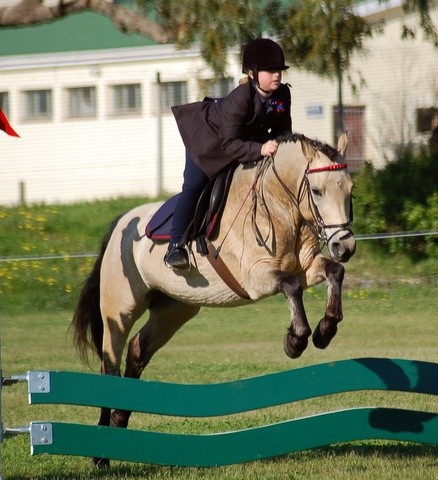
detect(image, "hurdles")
[1,357,438,480]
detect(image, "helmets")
[243,38,289,71]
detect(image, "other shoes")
[163,251,188,268]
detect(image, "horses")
[69,129,358,469]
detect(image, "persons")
[428,115,438,147]
[163,38,293,268]
